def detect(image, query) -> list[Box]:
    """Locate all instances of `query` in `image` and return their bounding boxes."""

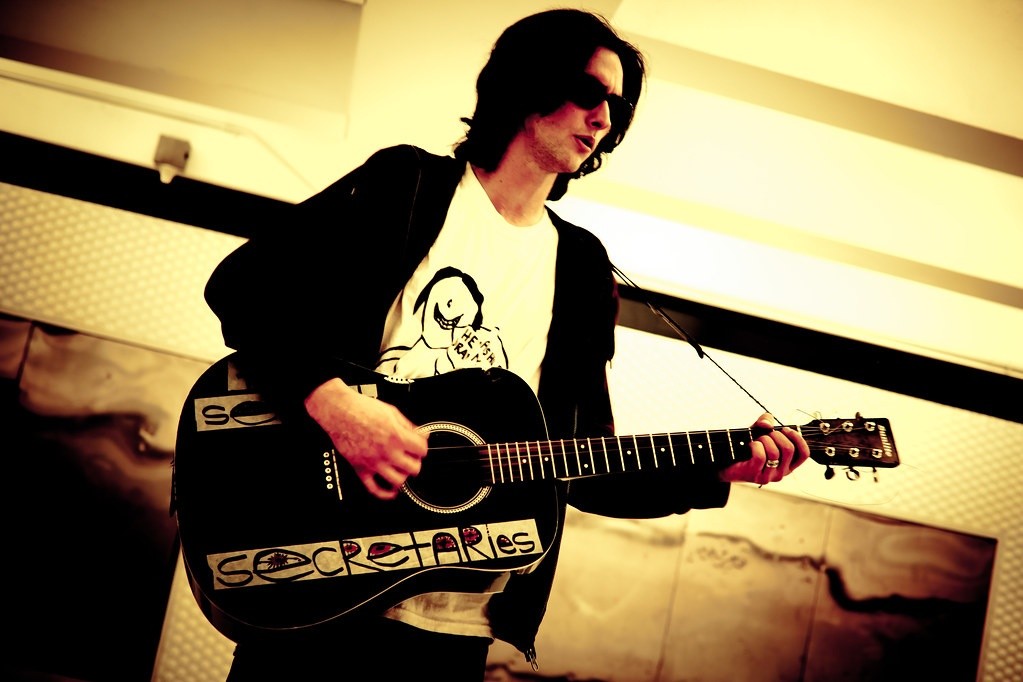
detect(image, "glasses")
[566,72,635,134]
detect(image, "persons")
[203,7,811,682]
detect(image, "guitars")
[174,349,902,653]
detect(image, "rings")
[766,460,779,468]
